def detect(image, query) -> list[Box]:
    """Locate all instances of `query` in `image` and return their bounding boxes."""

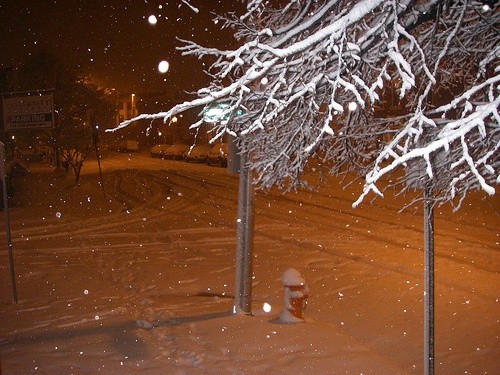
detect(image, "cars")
[14,165,31,175]
[108,140,205,163]
[206,148,228,168]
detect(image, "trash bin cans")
[6,169,32,208]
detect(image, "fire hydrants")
[283,269,309,321]
